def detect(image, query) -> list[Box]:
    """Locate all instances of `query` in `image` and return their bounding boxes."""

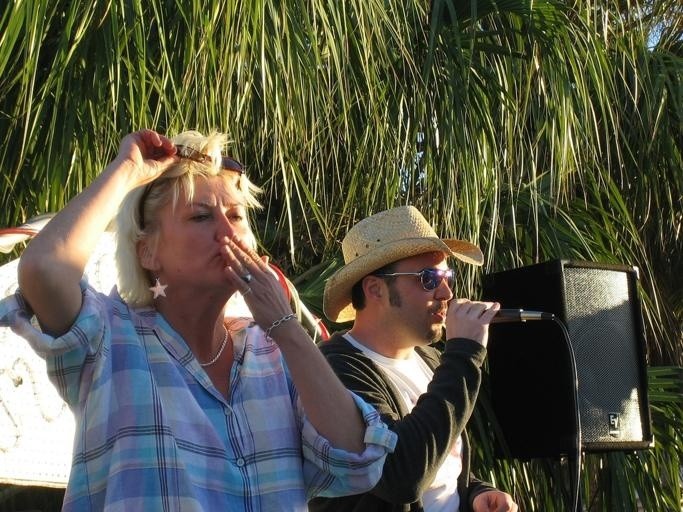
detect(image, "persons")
[0,125,402,511]
[300,204,524,512]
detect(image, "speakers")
[482,256,655,457]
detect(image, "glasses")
[368,269,457,294]
[138,144,246,229]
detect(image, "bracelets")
[265,312,300,346]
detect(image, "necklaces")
[197,321,228,370]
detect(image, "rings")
[239,287,255,299]
[243,271,257,284]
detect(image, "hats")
[323,205,486,324]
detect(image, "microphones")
[482,308,555,325]
